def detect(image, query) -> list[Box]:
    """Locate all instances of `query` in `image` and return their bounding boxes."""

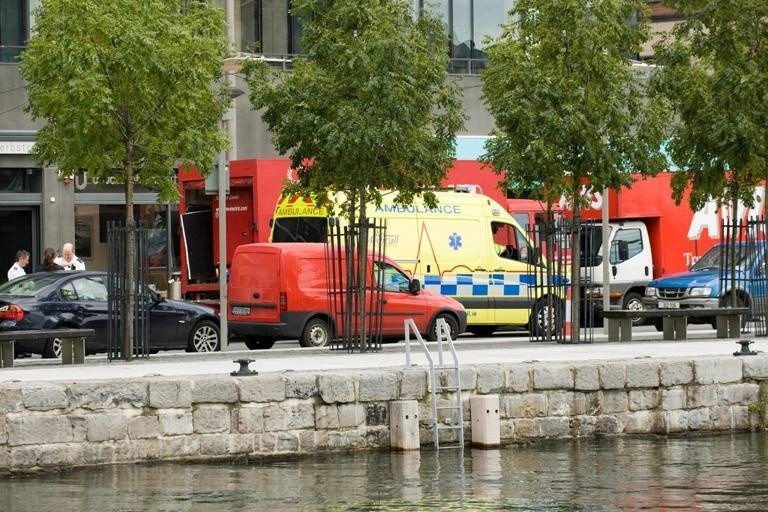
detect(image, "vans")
[227,241,469,348]
[268,184,570,337]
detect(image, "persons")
[7,249,32,358]
[35,242,85,272]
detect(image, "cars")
[640,240,766,332]
[0,268,221,358]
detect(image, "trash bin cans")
[168,271,182,300]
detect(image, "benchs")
[598,306,750,341]
[0,329,94,368]
